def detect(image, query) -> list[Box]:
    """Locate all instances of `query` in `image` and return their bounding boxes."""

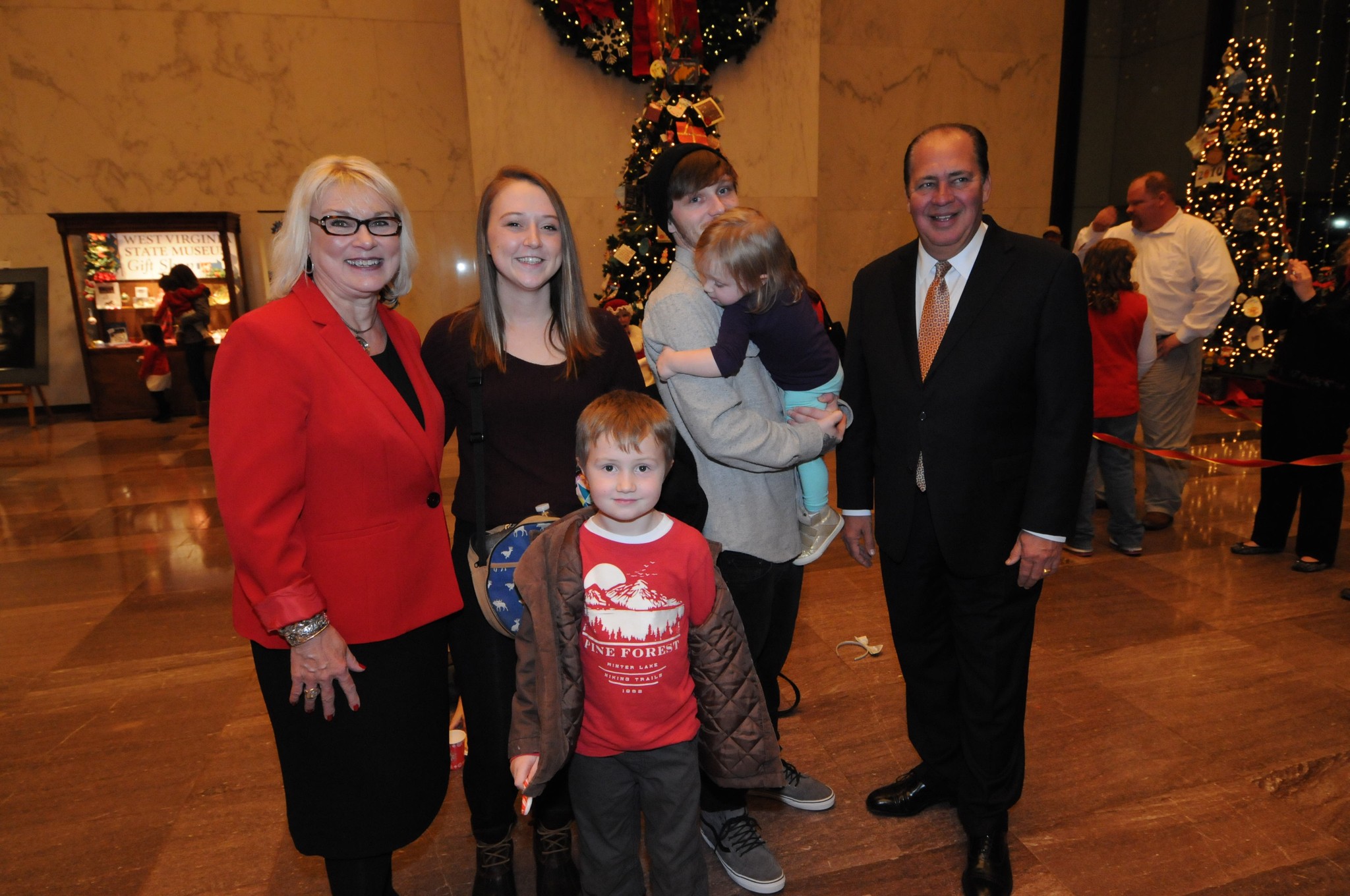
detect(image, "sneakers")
[748,759,836,811]
[700,808,785,892]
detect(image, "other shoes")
[1062,535,1094,557]
[471,833,516,896]
[1095,485,1112,503]
[1142,510,1173,531]
[1293,556,1325,571]
[1108,533,1143,555]
[1230,539,1274,555]
[534,822,582,895]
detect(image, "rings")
[1043,569,1052,575]
[304,687,320,699]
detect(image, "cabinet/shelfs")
[48,211,250,423]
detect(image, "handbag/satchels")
[467,516,561,638]
[823,321,847,355]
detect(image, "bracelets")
[279,609,330,646]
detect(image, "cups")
[449,730,466,770]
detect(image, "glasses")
[310,215,404,237]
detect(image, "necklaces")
[340,310,378,353]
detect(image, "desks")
[0,383,51,428]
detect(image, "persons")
[208,154,463,896]
[1230,238,1350,573]
[656,207,845,567]
[419,166,646,896]
[136,264,214,421]
[1063,238,1148,556]
[1072,171,1240,532]
[836,121,1092,896]
[642,141,853,896]
[506,390,785,896]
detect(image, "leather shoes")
[866,770,950,815]
[961,832,1015,895]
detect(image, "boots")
[190,401,209,428]
[153,403,173,423]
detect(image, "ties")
[918,260,951,382]
